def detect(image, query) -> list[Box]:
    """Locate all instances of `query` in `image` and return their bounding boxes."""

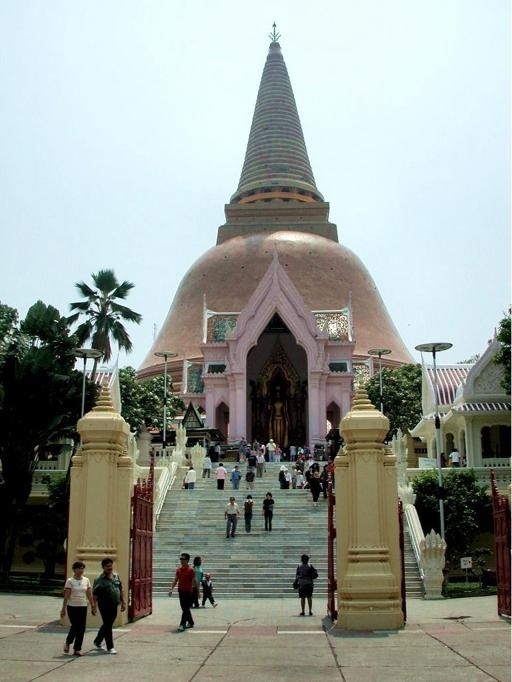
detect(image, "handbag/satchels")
[293,576,300,589]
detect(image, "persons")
[201,572,218,609]
[262,491,275,532]
[266,384,289,450]
[168,552,201,632]
[242,495,255,532]
[224,496,240,539]
[295,554,318,616]
[440,452,447,467]
[61,561,96,656]
[92,558,126,654]
[448,448,462,467]
[190,556,205,609]
[180,434,337,507]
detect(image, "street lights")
[66,346,106,452]
[366,346,393,416]
[153,350,179,450]
[413,340,453,538]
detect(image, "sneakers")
[63,640,86,656]
[177,621,194,631]
[299,610,313,616]
[93,639,117,654]
[212,603,218,608]
[194,602,206,607]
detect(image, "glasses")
[179,558,189,561]
